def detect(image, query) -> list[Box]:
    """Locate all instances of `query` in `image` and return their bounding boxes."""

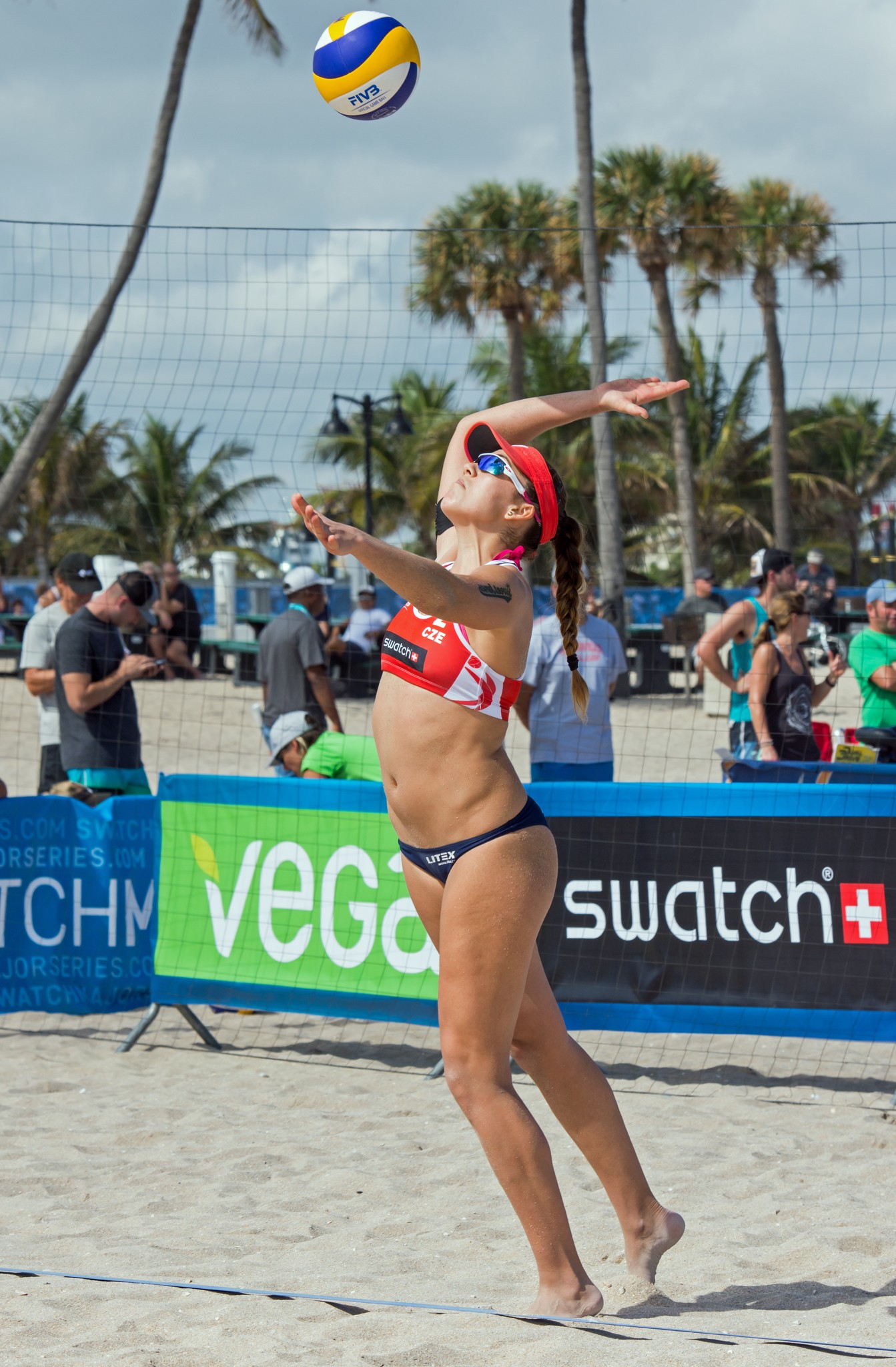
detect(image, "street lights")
[316,392,417,587]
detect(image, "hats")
[282,565,335,595]
[806,549,825,564]
[694,565,715,581]
[264,709,318,766]
[866,578,896,603]
[741,547,793,588]
[58,552,103,594]
[463,421,558,545]
[118,570,154,606]
[357,584,376,596]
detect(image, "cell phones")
[154,658,168,665]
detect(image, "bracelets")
[760,739,773,748]
[826,677,836,688]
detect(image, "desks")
[241,615,347,642]
[629,623,677,694]
[834,610,869,633]
[0,613,33,641]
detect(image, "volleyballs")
[312,9,422,121]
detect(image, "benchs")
[0,641,23,674]
[199,640,260,684]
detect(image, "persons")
[291,376,689,1319]
[0,560,391,795]
[676,547,896,762]
[513,556,629,782]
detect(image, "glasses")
[474,453,541,524]
[802,609,813,615]
